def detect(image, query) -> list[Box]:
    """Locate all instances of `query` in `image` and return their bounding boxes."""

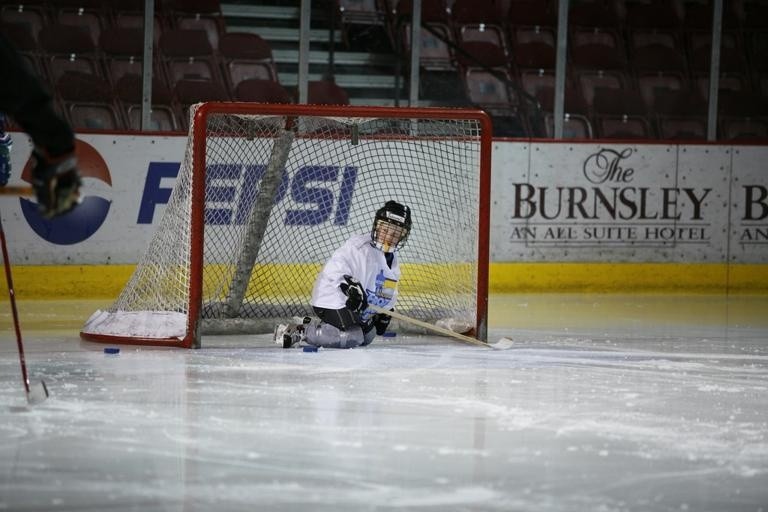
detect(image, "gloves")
[32,152,78,220]
[376,308,394,335]
[341,274,369,314]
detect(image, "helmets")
[370,200,412,254]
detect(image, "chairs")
[1,0,767,146]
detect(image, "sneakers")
[283,326,306,348]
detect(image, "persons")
[272,200,413,350]
[0,26,80,219]
